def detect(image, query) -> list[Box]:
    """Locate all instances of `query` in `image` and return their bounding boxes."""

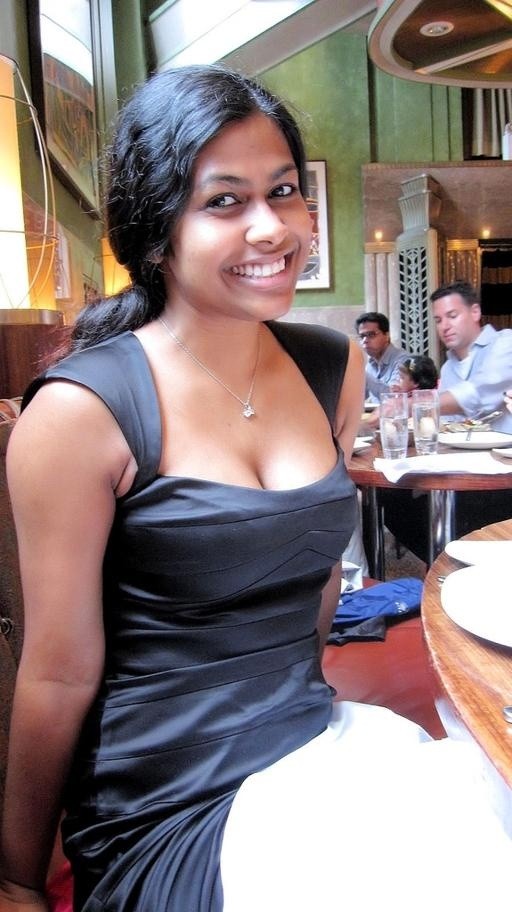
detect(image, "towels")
[372,452,512,487]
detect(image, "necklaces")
[157,314,279,419]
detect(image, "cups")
[412,390,440,453]
[379,392,409,460]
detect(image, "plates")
[441,563,512,649]
[439,431,512,451]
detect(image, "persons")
[384,353,441,402]
[354,309,408,405]
[1,62,511,907]
[364,278,512,573]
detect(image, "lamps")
[24,231,59,314]
[100,235,132,300]
[1,58,64,396]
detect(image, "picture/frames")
[294,160,335,290]
[25,0,103,219]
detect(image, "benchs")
[1,413,446,912]
